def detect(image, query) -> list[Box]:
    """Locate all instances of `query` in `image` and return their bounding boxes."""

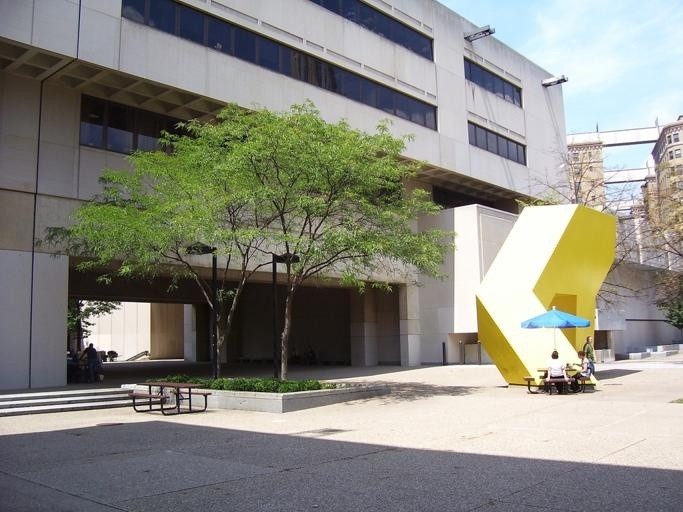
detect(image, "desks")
[135,381,205,416]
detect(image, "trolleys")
[66,357,97,383]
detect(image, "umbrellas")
[521,305,591,351]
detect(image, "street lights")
[183,242,218,379]
[269,250,303,381]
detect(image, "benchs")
[519,365,595,395]
[168,389,212,413]
[126,391,172,417]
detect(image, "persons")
[303,344,315,366]
[571,351,591,393]
[582,336,596,376]
[81,343,98,383]
[549,351,569,394]
[291,347,300,360]
